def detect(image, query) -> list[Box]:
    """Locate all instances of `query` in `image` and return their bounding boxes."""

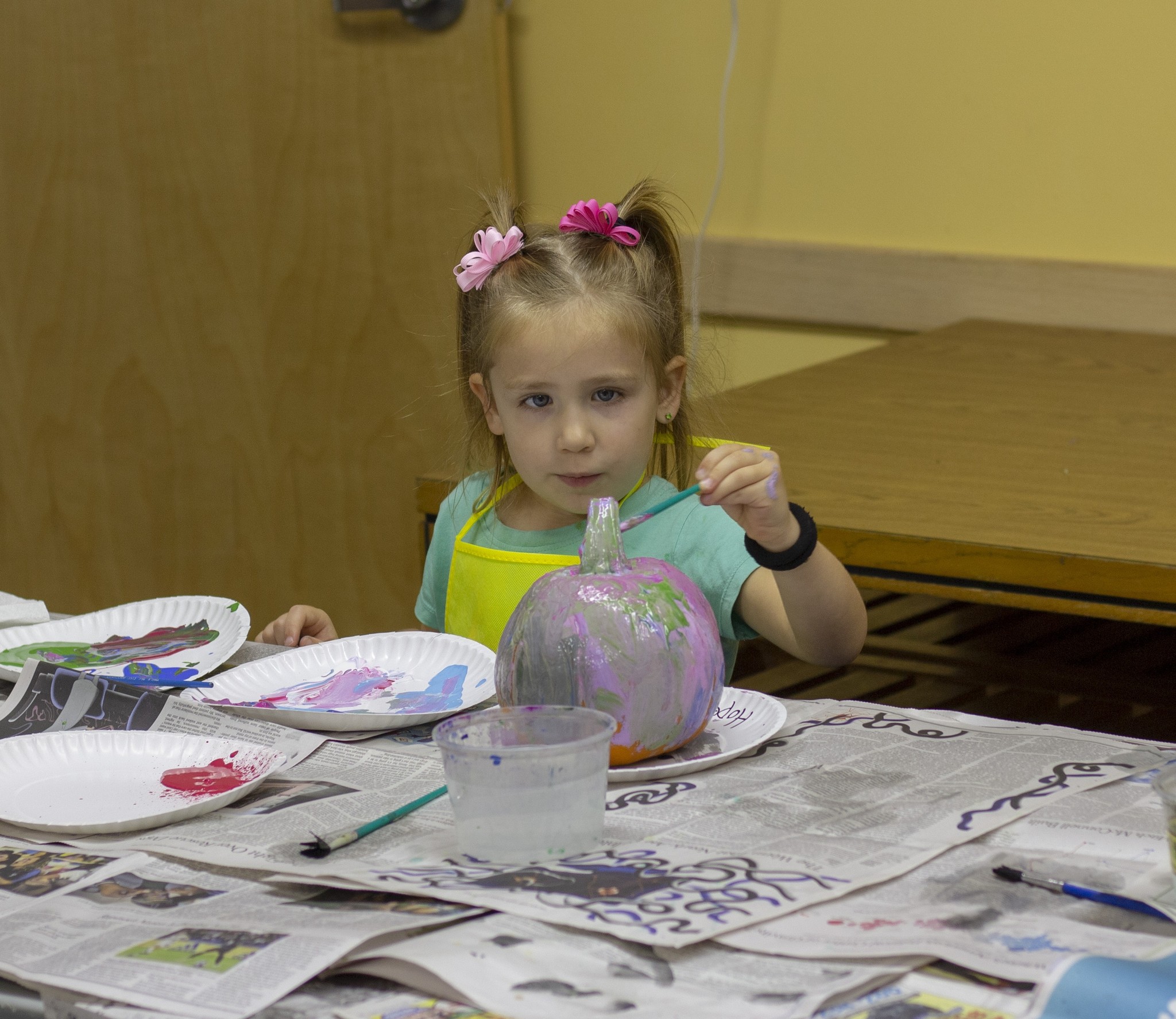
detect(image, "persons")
[243,175,868,691]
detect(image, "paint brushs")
[621,483,701,534]
[300,784,447,859]
[95,674,214,689]
[991,865,1173,924]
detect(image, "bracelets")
[744,500,820,573]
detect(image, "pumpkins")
[494,496,726,767]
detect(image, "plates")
[179,631,499,730]
[482,686,787,782]
[1,730,286,836]
[0,596,250,692]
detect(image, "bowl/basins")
[431,703,621,859]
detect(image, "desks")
[413,312,1176,634]
